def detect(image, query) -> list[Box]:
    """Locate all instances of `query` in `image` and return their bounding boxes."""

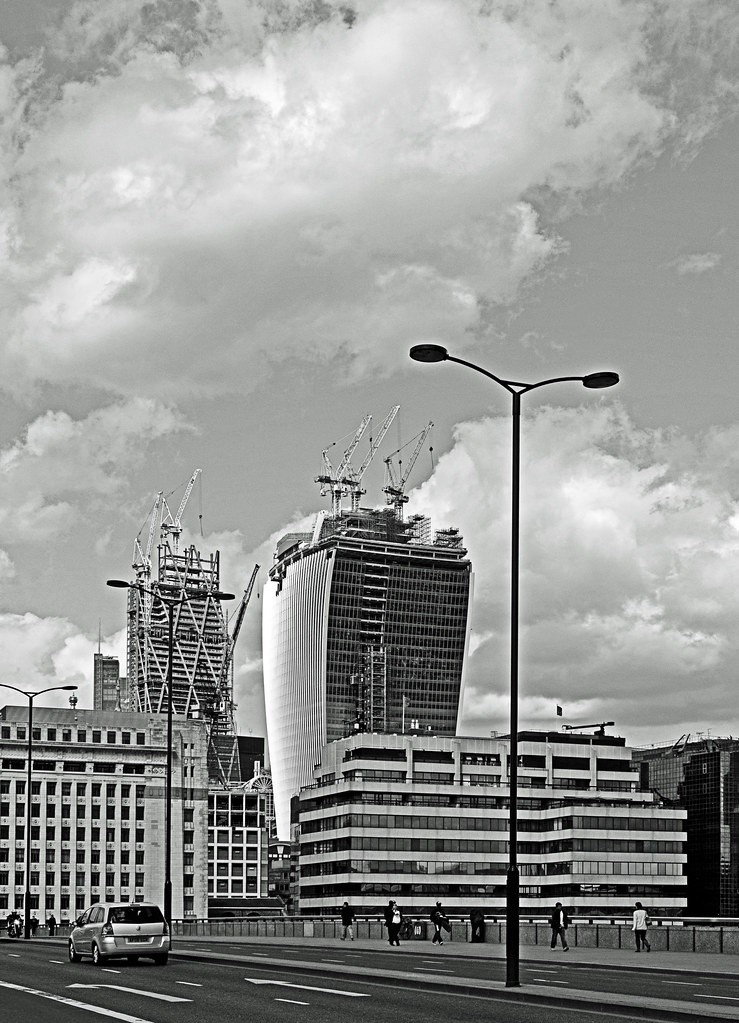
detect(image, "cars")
[68,900,171,967]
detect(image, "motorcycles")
[4,914,25,939]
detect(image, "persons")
[48,915,56,936]
[30,916,39,938]
[470,910,486,944]
[429,901,448,945]
[632,902,652,953]
[384,900,401,946]
[340,902,355,941]
[549,902,570,952]
[7,908,23,935]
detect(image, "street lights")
[0,680,79,943]
[409,341,625,988]
[106,579,240,955]
